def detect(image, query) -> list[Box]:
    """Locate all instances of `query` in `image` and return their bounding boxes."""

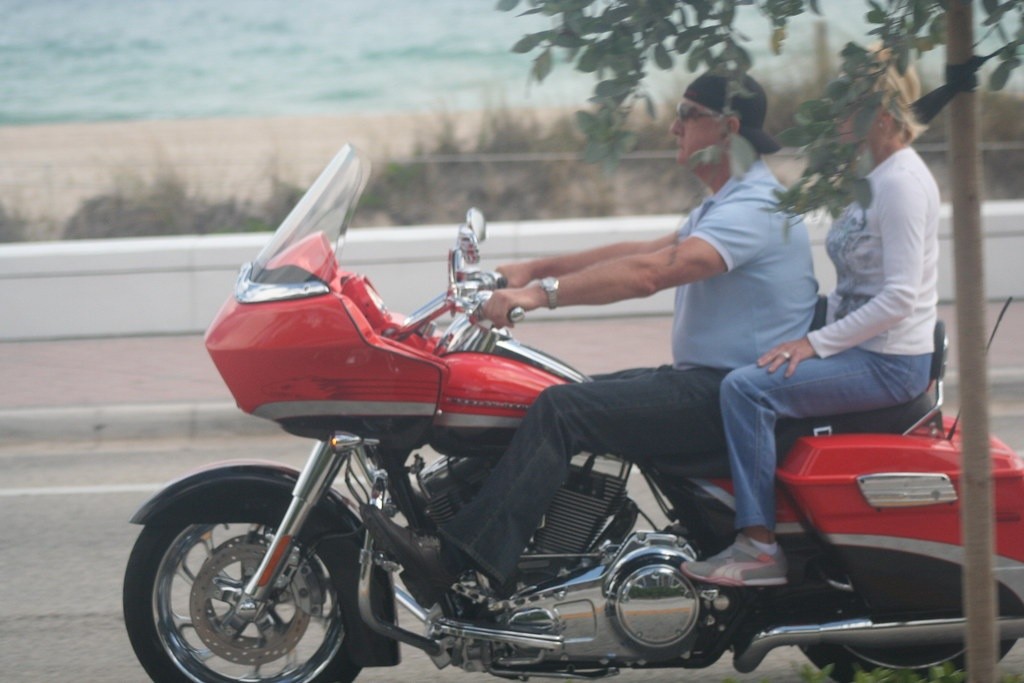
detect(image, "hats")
[684,67,780,152]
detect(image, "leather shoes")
[364,504,455,607]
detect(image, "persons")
[679,41,940,586]
[361,69,818,609]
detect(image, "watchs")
[539,275,559,311]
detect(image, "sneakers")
[681,534,791,586]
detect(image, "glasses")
[677,101,720,121]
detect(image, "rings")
[780,350,791,362]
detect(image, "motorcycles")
[118,140,1024,682]
